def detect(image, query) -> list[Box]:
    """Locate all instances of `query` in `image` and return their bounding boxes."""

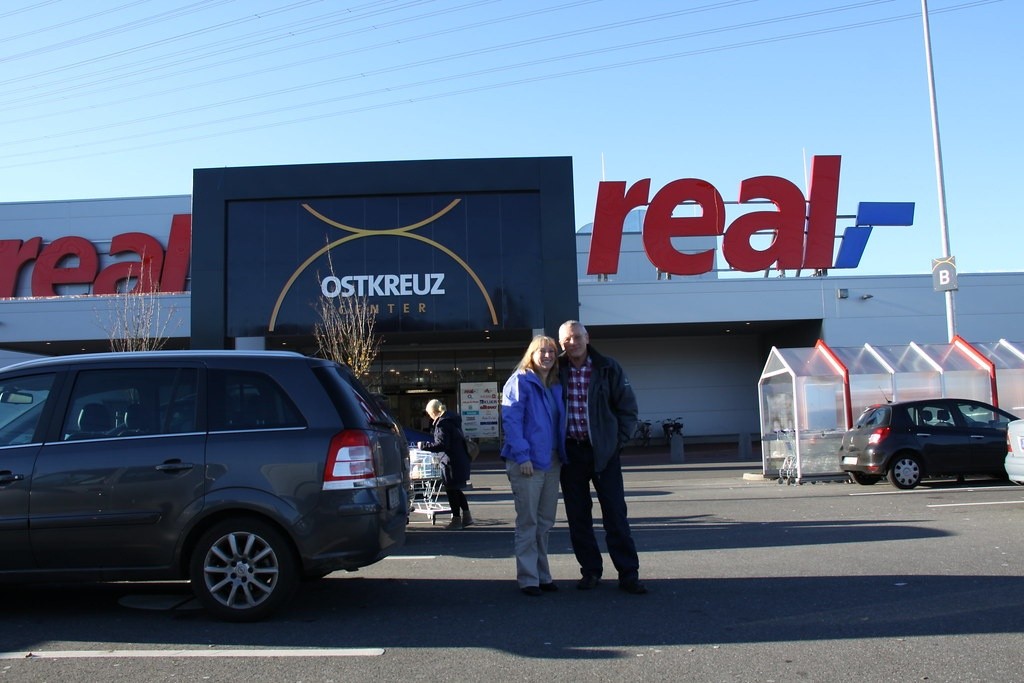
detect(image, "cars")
[838,398,1021,490]
[1,349,413,623]
[1004,419,1024,487]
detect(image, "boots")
[461,511,473,527]
[445,517,463,530]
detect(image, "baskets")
[636,423,645,429]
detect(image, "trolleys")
[406,442,453,525]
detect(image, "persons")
[417,399,474,531]
[557,320,652,595]
[499,336,569,598]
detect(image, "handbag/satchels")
[465,437,479,461]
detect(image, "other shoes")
[520,586,539,596]
[539,583,558,592]
[618,578,642,598]
[576,574,604,590]
